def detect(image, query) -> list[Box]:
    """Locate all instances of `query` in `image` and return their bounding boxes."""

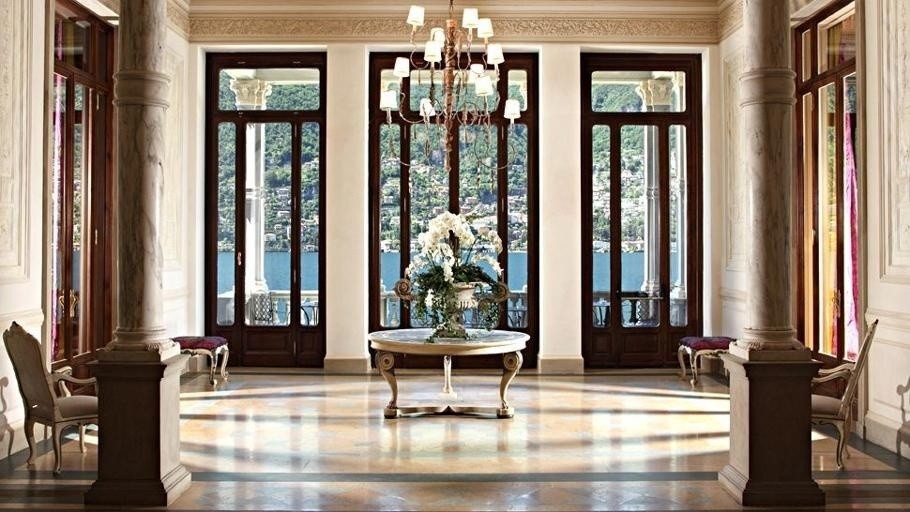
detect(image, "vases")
[425,280,478,343]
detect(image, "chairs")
[2,320,98,479]
[789,318,881,472]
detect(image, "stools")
[172,335,231,386]
[677,334,737,390]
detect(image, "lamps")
[379,2,525,176]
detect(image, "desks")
[367,327,532,423]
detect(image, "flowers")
[404,210,505,326]
